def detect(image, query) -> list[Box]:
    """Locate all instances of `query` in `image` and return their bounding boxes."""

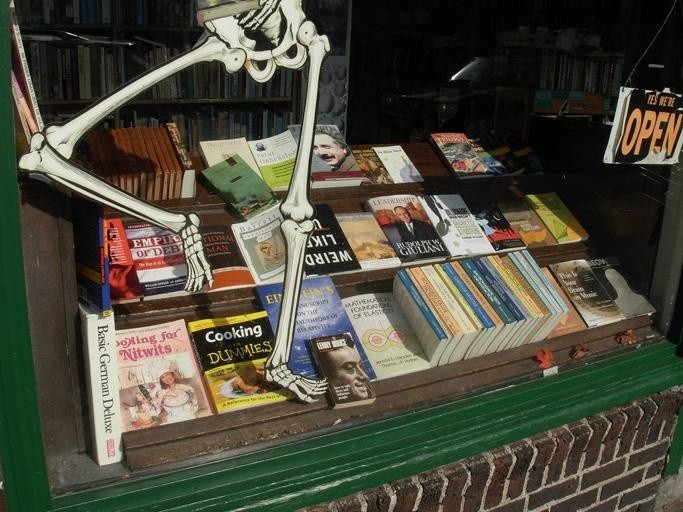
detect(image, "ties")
[409,225,415,237]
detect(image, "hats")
[158,367,175,390]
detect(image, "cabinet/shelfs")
[14,1,301,139]
[494,45,624,142]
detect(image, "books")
[10,0,656,466]
